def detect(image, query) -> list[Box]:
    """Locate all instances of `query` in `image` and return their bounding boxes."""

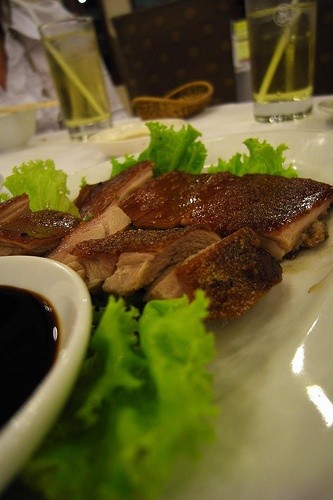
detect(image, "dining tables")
[1,95,332,495]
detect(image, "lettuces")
[0,289,223,500]
[0,120,297,217]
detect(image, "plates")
[0,131,333,499]
[0,255,93,492]
[317,97,333,114]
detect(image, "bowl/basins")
[87,118,185,157]
[0,102,36,152]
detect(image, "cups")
[38,16,112,142]
[246,0,317,124]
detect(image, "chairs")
[110,0,238,106]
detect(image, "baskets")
[132,81,213,122]
